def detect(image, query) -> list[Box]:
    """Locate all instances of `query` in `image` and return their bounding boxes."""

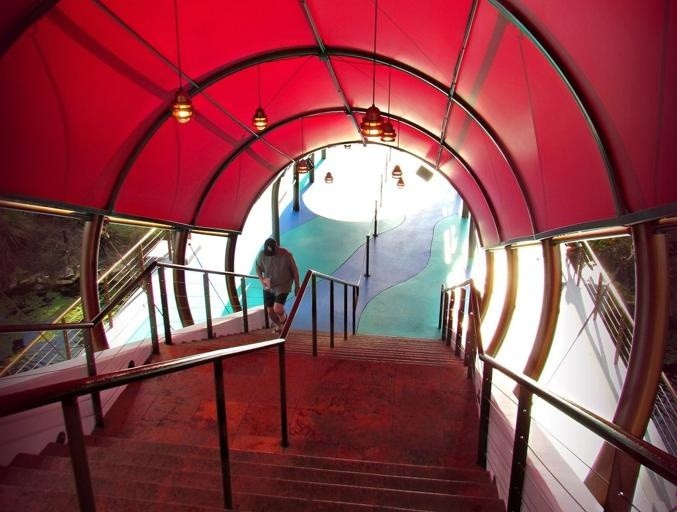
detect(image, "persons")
[255,239,300,334]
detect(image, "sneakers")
[273,328,281,338]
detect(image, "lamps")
[325,171,333,183]
[398,178,405,189]
[392,111,403,179]
[252,64,268,131]
[381,56,396,142]
[361,0,383,137]
[296,115,308,174]
[170,1,193,123]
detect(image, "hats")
[264,238,277,255]
